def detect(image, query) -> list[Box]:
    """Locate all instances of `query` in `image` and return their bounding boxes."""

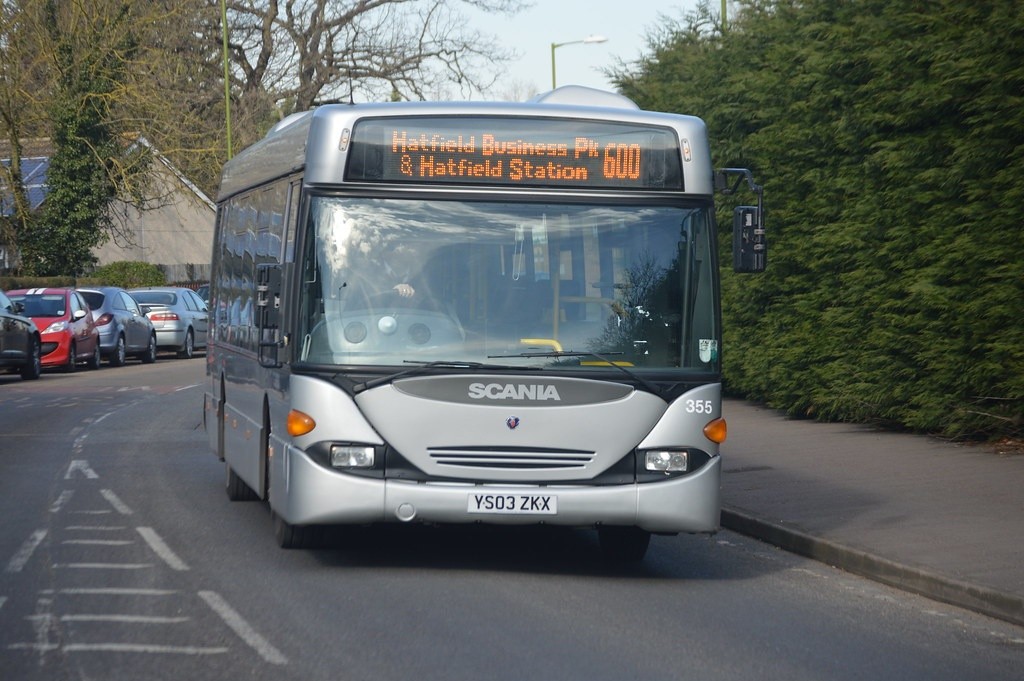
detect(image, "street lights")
[550,35,611,90]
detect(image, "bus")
[203,85,769,560]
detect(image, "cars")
[67,286,158,368]
[0,287,44,380]
[4,288,101,373]
[124,284,211,358]
[192,283,211,310]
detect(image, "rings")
[403,290,406,292]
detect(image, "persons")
[342,252,430,310]
